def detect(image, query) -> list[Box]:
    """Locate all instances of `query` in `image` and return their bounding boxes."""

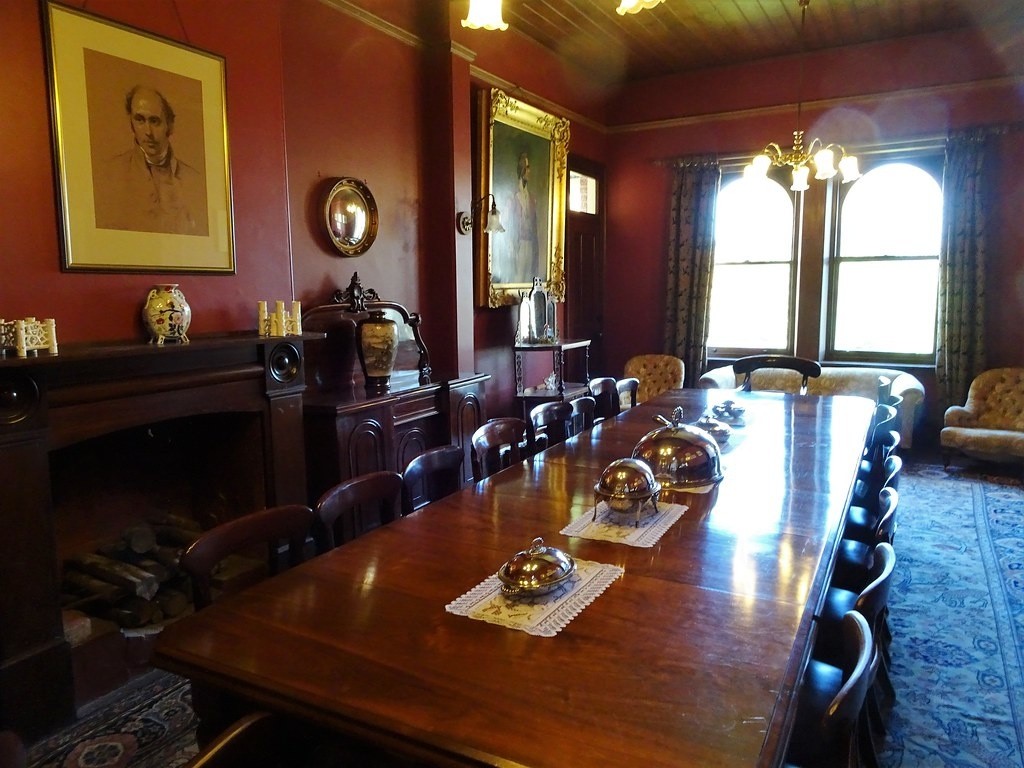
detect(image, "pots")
[652,414,733,443]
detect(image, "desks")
[150,387,876,768]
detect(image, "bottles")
[142,283,191,344]
[355,310,399,389]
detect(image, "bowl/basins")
[592,458,659,528]
[712,401,745,417]
[498,537,575,596]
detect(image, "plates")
[629,406,724,489]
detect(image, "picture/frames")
[474,87,570,311]
[323,176,379,259]
[36,0,238,277]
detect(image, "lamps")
[457,194,506,235]
[616,0,665,16]
[742,1,864,193]
[461,0,510,32]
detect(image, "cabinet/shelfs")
[300,271,494,544]
[513,275,593,421]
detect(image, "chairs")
[175,377,644,768]
[733,355,905,768]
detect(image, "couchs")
[619,354,686,412]
[937,367,1024,470]
[699,364,927,464]
